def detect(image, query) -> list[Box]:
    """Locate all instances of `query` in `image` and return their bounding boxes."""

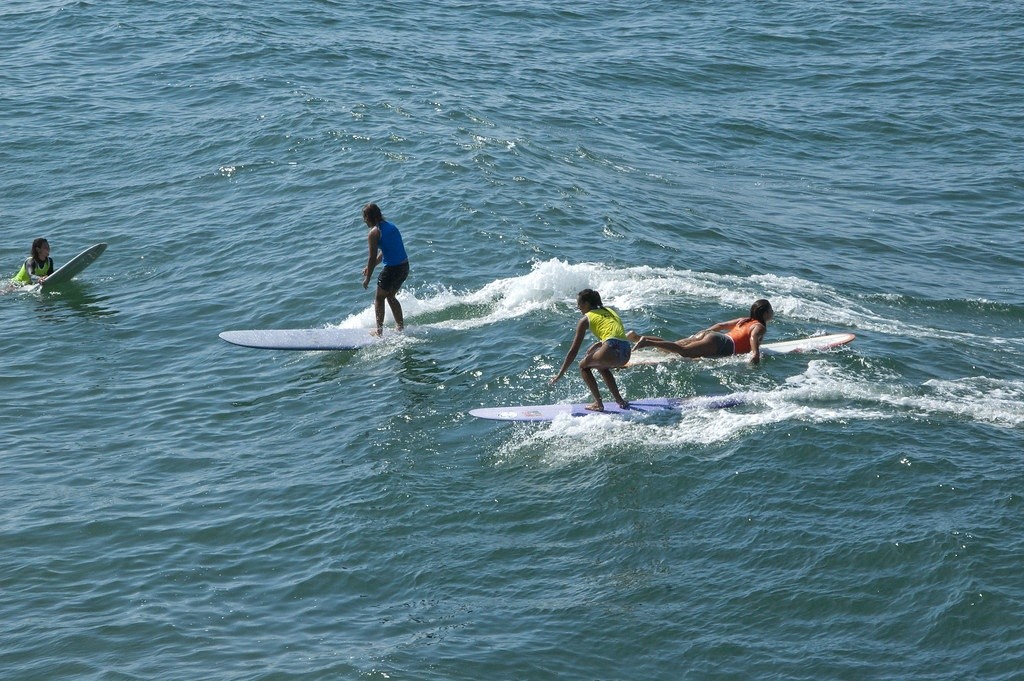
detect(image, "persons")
[551,287,631,410]
[624,298,774,364]
[10,238,54,284]
[362,203,410,338]
